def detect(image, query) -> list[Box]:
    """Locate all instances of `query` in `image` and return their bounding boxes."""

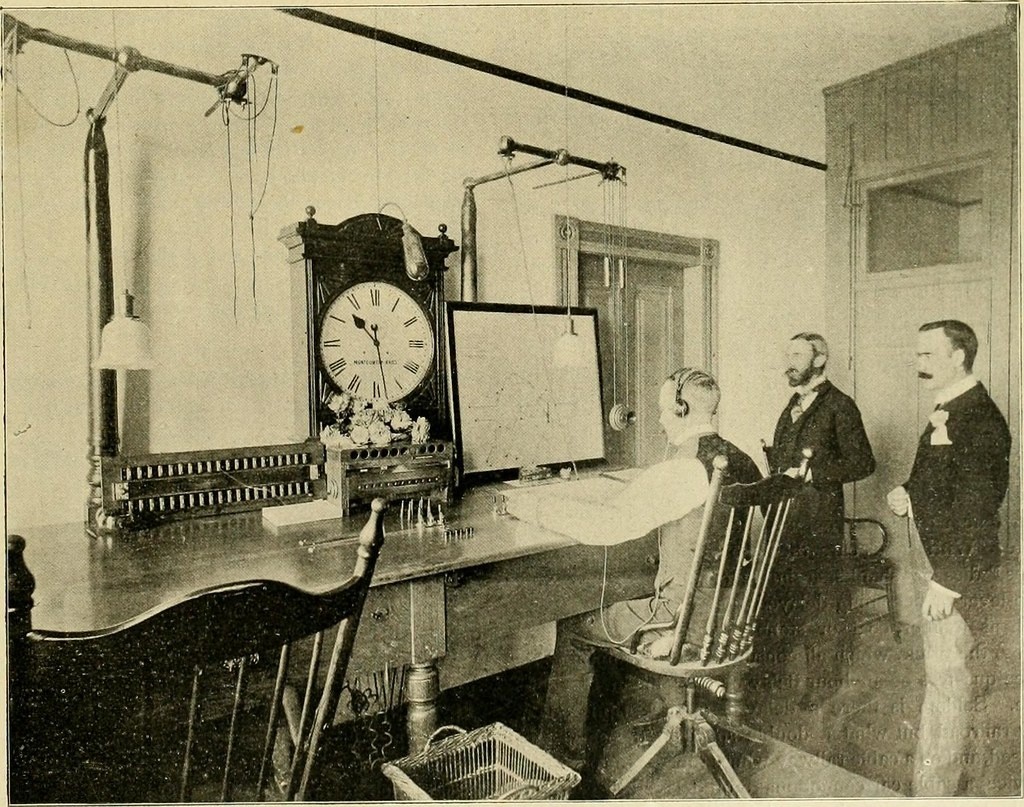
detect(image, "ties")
[789,394,807,425]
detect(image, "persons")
[887,321,1013,798]
[734,330,879,712]
[501,366,766,781]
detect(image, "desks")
[7,463,664,721]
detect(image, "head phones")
[674,366,720,417]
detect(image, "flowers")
[319,394,431,449]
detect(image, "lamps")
[91,9,156,371]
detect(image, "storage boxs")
[381,719,582,802]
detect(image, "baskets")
[380,722,582,804]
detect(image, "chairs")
[7,495,388,803]
[837,517,902,667]
[567,446,819,797]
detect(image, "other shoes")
[629,722,682,758]
[796,673,843,711]
[566,766,591,801]
[743,674,792,696]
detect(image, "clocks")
[277,212,460,436]
